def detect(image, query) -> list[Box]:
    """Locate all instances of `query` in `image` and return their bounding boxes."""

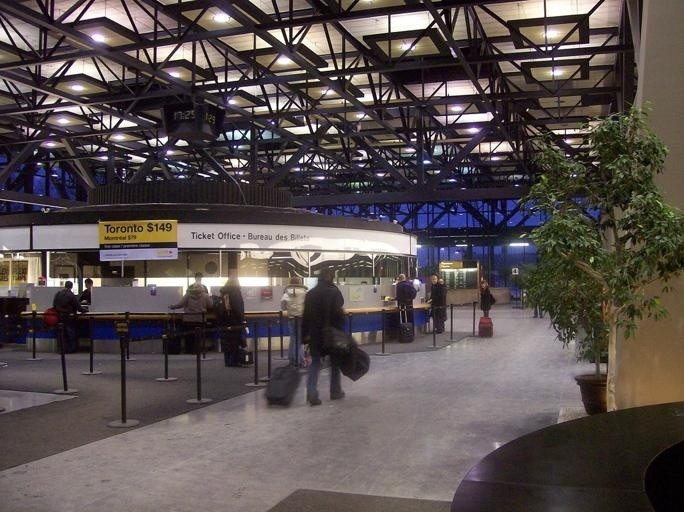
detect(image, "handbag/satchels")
[489,294,496,306]
[41,306,60,326]
[339,340,372,381]
[236,344,253,365]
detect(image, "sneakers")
[307,387,347,406]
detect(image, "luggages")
[478,316,493,338]
[398,304,414,343]
[263,364,302,407]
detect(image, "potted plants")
[478,288,496,336]
[510,100,684,417]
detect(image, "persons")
[425,273,448,334]
[280,275,310,369]
[77,277,93,304]
[300,266,344,406]
[53,281,88,355]
[388,273,417,337]
[168,273,248,368]
[479,280,491,317]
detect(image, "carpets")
[0,331,476,471]
[267,488,452,512]
